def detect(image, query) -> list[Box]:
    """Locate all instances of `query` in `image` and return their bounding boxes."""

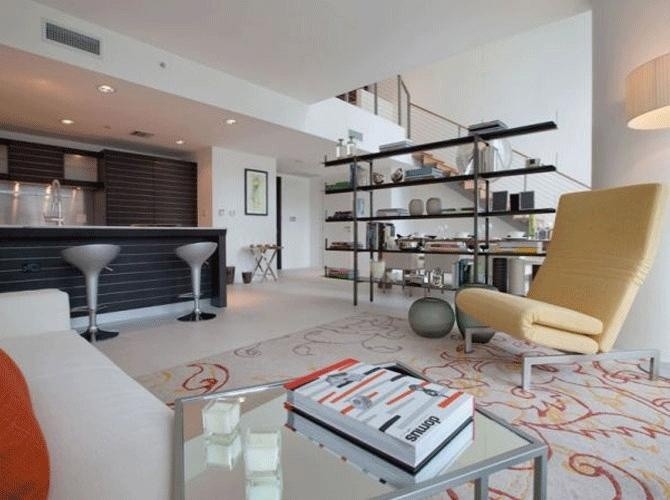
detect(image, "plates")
[426,197,443,215]
[407,198,425,218]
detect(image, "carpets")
[127,305,670,500]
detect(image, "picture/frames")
[243,167,269,217]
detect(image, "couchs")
[1,287,175,500]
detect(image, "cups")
[201,397,242,448]
[202,437,242,474]
[243,476,283,499]
[244,426,281,482]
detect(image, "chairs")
[457,181,669,390]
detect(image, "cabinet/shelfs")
[320,116,564,309]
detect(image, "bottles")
[347,134,357,158]
[335,138,345,161]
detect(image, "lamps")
[622,51,670,132]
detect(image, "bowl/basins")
[394,234,422,251]
[508,231,526,238]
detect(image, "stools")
[61,242,123,345]
[174,238,219,323]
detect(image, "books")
[327,269,354,279]
[282,358,477,488]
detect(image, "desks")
[242,245,283,283]
